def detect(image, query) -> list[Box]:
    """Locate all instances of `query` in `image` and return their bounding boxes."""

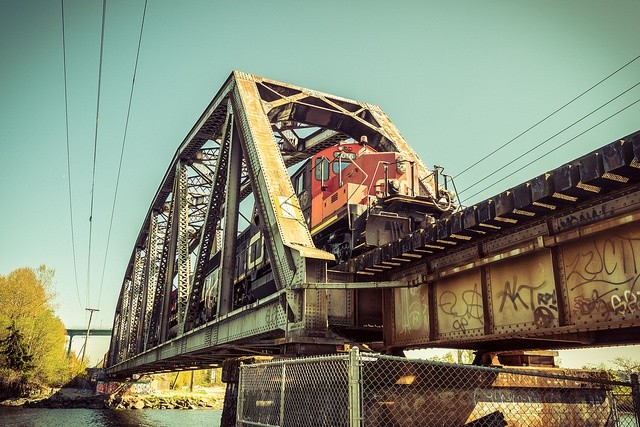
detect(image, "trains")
[166,135,456,337]
